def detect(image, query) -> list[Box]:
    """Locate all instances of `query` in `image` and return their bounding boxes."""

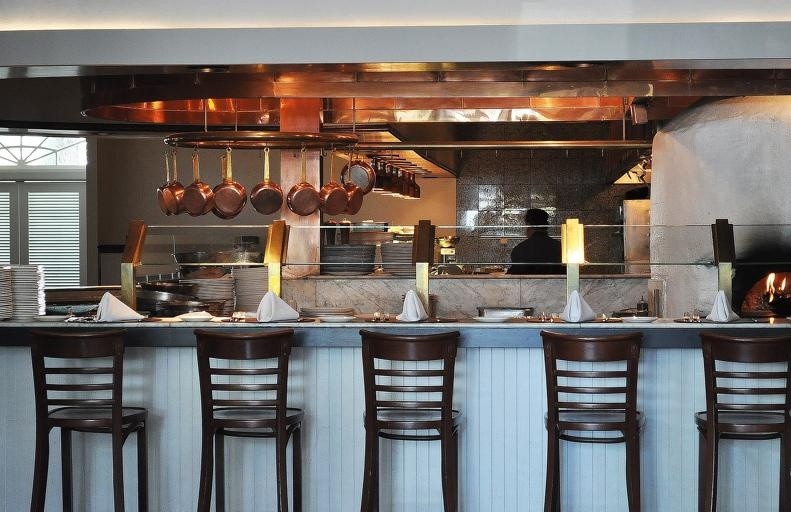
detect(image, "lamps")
[365,153,431,200]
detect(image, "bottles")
[636,296,648,317]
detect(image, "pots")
[154,138,376,220]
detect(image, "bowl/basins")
[169,248,263,278]
[136,279,227,316]
[434,234,461,248]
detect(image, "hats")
[526,208,550,225]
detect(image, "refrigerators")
[622,200,652,275]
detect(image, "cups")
[647,278,664,319]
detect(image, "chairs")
[695,333,790,512]
[30,329,149,512]
[537,330,645,512]
[359,329,462,512]
[193,328,304,512]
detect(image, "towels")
[95,291,148,322]
[395,289,429,323]
[706,290,741,322]
[559,289,597,322]
[256,290,299,321]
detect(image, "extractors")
[366,153,431,179]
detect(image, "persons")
[506,209,567,275]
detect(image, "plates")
[180,316,215,322]
[297,305,357,322]
[320,240,416,277]
[177,265,269,315]
[0,262,46,322]
[620,316,658,324]
[472,315,513,323]
[33,315,70,322]
[46,304,98,315]
